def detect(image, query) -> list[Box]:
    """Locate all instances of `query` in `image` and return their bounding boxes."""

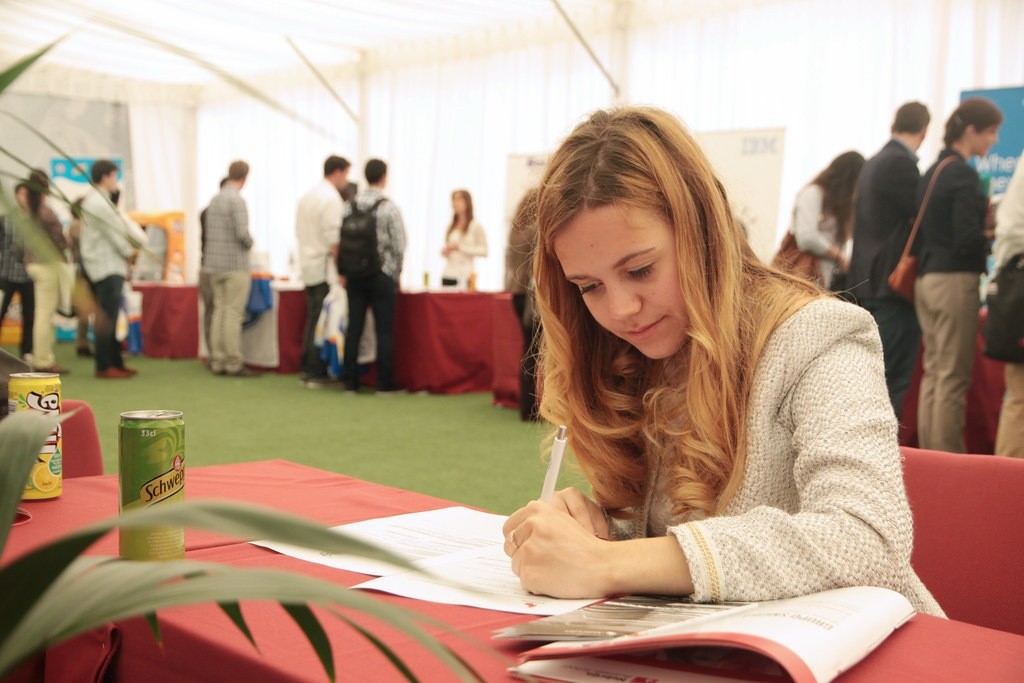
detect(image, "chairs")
[897,446,1024,637]
[60,399,103,478]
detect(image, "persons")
[502,107,946,620]
[0,170,69,373]
[506,188,544,419]
[70,160,147,378]
[850,103,931,419]
[772,150,866,291]
[914,99,1003,453]
[199,161,259,376]
[441,190,488,287]
[296,155,406,395]
[992,154,1024,459]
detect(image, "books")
[492,586,916,683]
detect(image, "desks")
[896,309,1006,455]
[122,284,524,410]
[0,459,1024,683]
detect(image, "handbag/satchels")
[976,251,1024,363]
[887,255,918,308]
[771,232,826,291]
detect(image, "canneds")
[117,409,186,563]
[8,372,64,500]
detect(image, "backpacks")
[337,198,389,278]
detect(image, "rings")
[510,529,519,548]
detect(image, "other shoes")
[94,366,129,380]
[343,384,363,396]
[298,374,327,391]
[377,382,408,397]
[31,366,69,376]
[118,365,138,376]
[211,364,262,379]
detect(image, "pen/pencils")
[539,424,568,502]
